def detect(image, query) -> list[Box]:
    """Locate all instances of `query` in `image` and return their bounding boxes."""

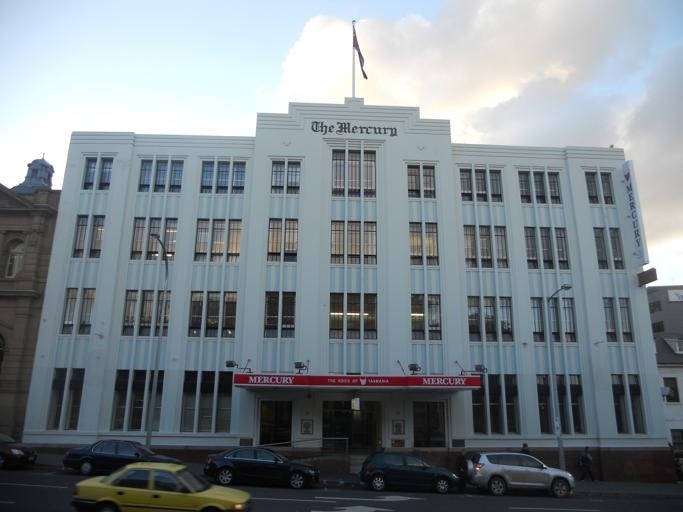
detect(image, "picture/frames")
[301,419,313,434]
[391,418,406,435]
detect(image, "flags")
[352,27,368,80]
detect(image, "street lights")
[548,284,572,469]
[146,234,168,448]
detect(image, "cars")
[0,433,38,464]
[466,452,576,498]
[71,462,254,512]
[204,447,320,489]
[359,452,460,493]
[64,440,182,474]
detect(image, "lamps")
[295,362,305,374]
[226,360,252,374]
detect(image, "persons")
[580,445,597,481]
[455,448,467,493]
[519,443,529,454]
